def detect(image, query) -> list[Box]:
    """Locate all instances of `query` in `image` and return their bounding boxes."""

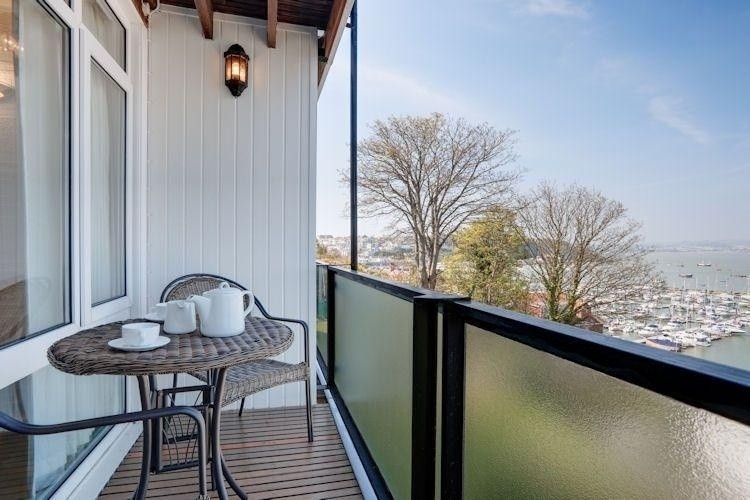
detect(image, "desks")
[48,317,293,499]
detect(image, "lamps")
[223,43,249,98]
[160,273,314,442]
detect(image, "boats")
[521,278,750,351]
[679,273,692,279]
[729,273,747,278]
[696,259,712,267]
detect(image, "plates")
[107,336,171,352]
[145,313,164,322]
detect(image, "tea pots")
[187,281,255,337]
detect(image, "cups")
[162,300,196,334]
[151,303,168,319]
[120,323,161,348]
